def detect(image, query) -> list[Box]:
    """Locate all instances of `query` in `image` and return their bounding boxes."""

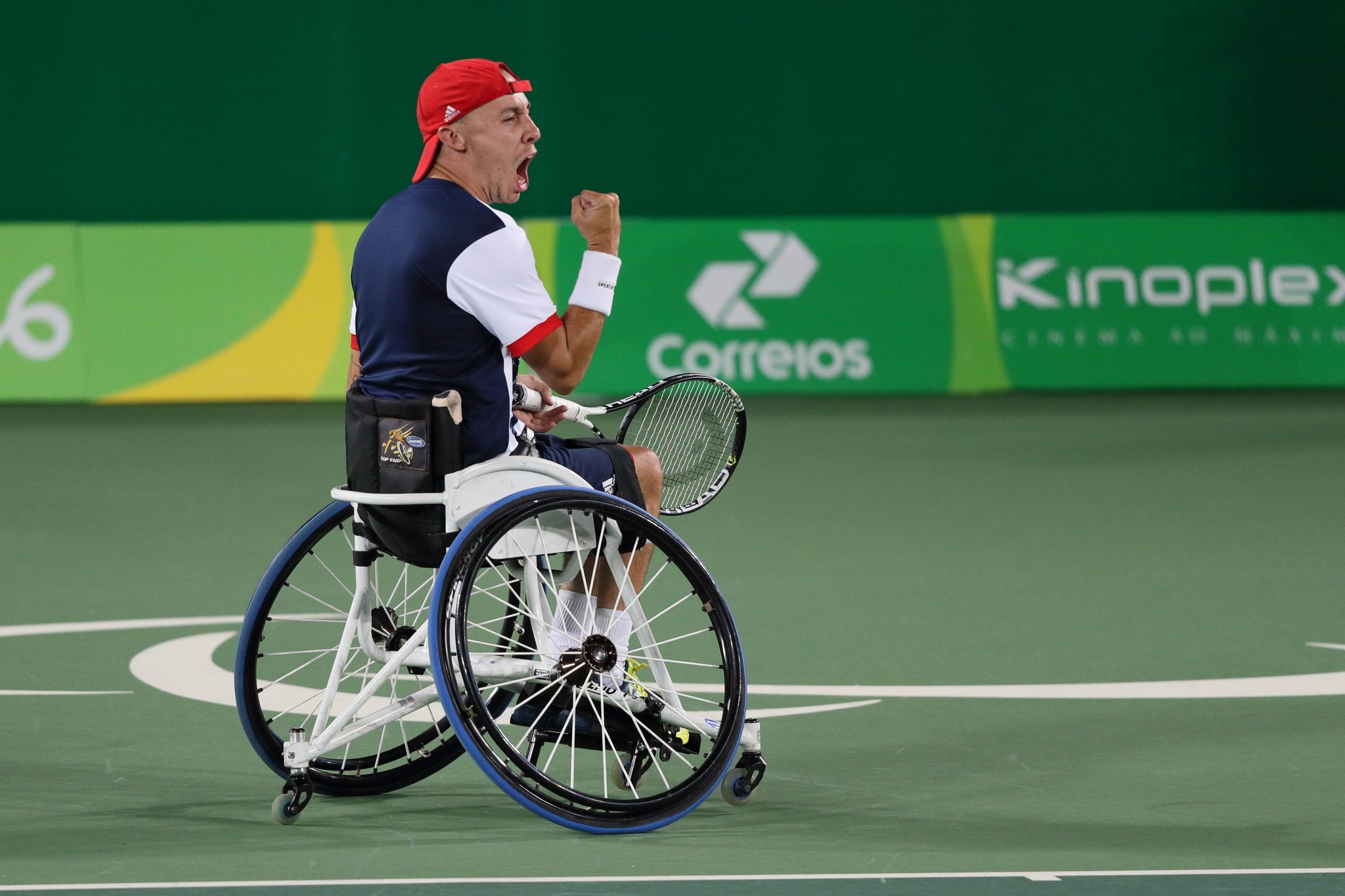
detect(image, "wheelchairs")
[235,389,769,835]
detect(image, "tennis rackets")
[513,371,750,518]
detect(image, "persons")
[347,59,664,715]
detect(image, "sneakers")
[509,682,572,729]
[556,657,690,745]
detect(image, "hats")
[412,59,532,184]
